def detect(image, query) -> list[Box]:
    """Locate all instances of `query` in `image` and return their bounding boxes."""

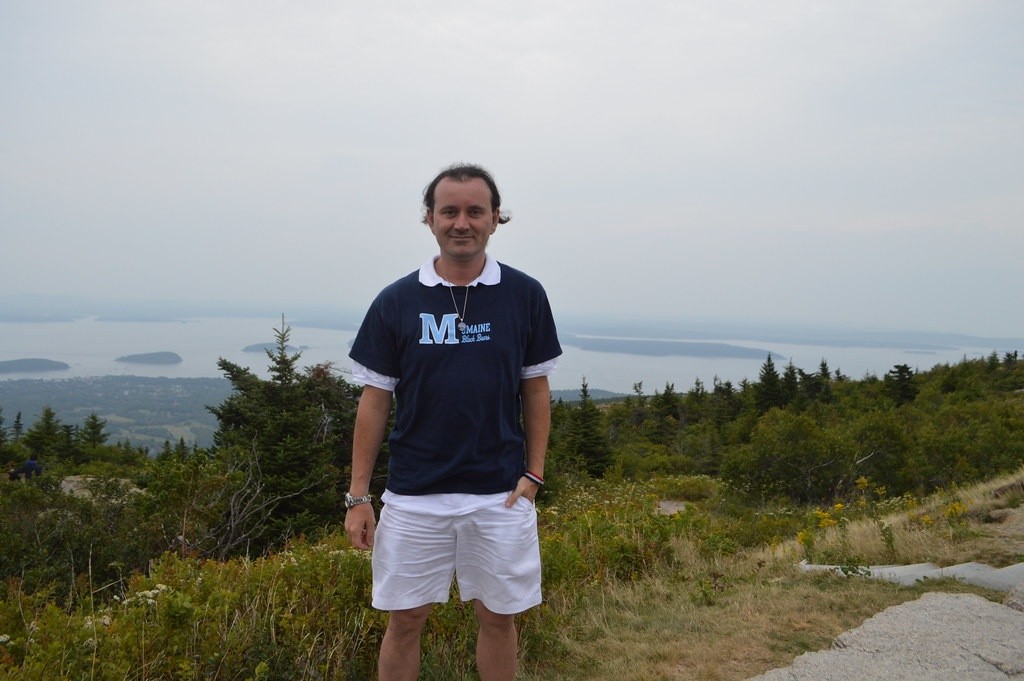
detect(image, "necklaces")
[443,272,480,331]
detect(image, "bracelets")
[523,470,545,486]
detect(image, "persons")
[344,165,564,681]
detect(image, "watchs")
[344,492,371,508]
